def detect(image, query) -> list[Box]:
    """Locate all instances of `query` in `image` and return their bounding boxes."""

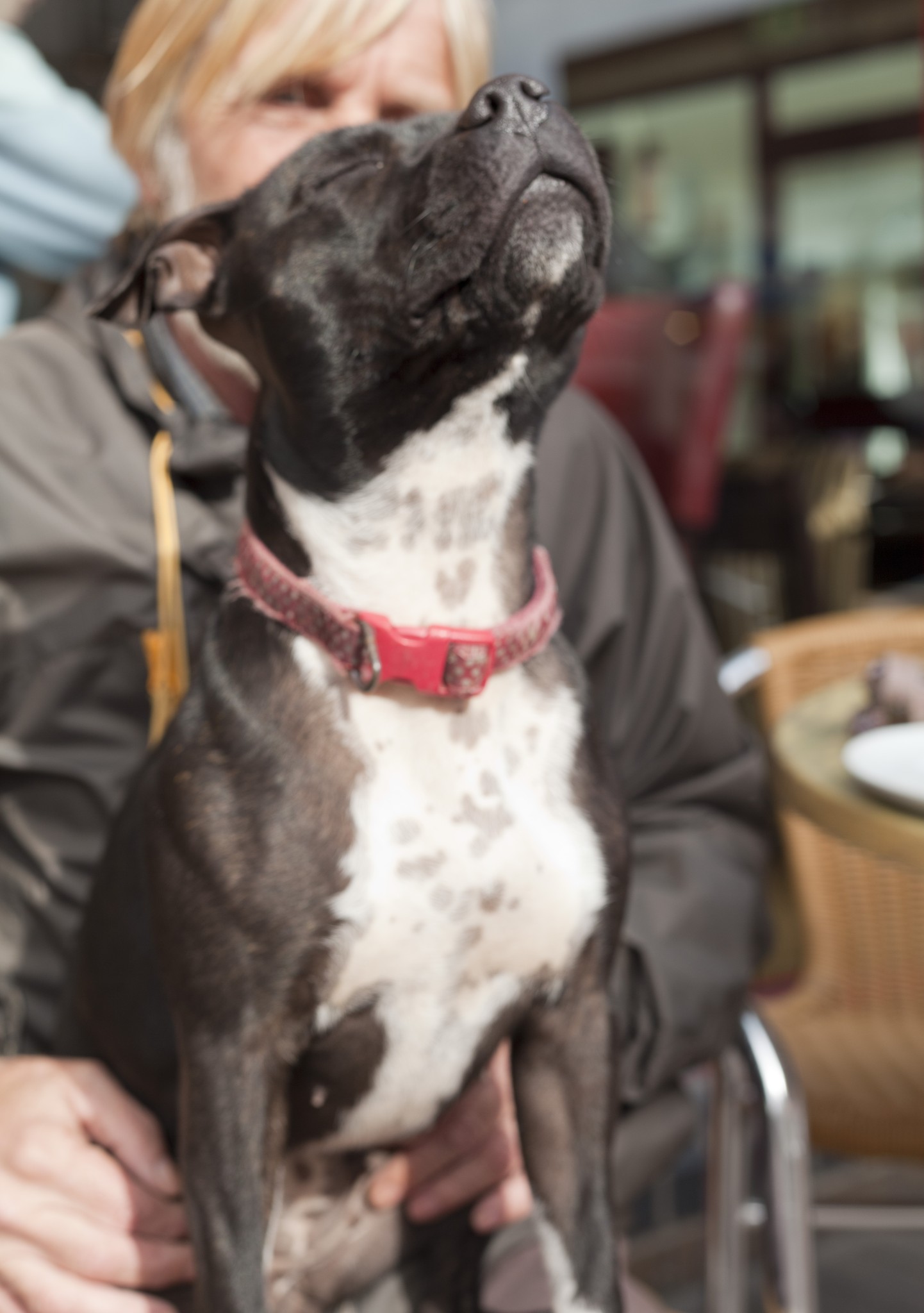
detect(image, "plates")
[837,720,924,818]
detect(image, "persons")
[0,0,794,1313]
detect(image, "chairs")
[701,603,924,1313]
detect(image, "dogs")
[50,70,627,1312]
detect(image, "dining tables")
[765,660,924,876]
